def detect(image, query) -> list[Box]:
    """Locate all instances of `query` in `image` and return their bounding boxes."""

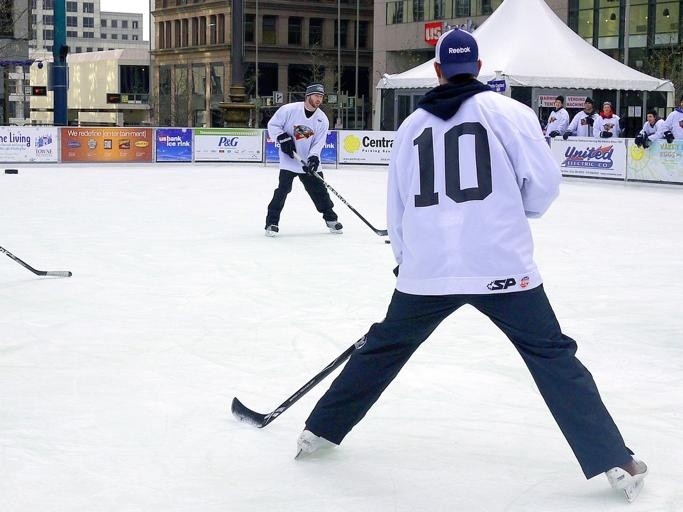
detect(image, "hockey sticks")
[293,149,387,236]
[0,247,72,277]
[232,336,365,430]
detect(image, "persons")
[635,109,665,148]
[663,97,683,143]
[563,97,599,140]
[592,101,626,137]
[544,96,570,137]
[299,25,645,492]
[264,82,343,231]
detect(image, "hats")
[436,28,478,78]
[585,97,593,103]
[556,95,564,104]
[306,83,326,96]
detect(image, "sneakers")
[266,225,280,233]
[326,221,343,229]
[615,457,648,479]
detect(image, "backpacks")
[585,117,593,125]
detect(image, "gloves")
[302,156,319,175]
[550,130,560,138]
[563,132,571,140]
[278,133,297,159]
[600,131,612,138]
[636,131,650,149]
[664,131,674,144]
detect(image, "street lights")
[23,59,44,119]
